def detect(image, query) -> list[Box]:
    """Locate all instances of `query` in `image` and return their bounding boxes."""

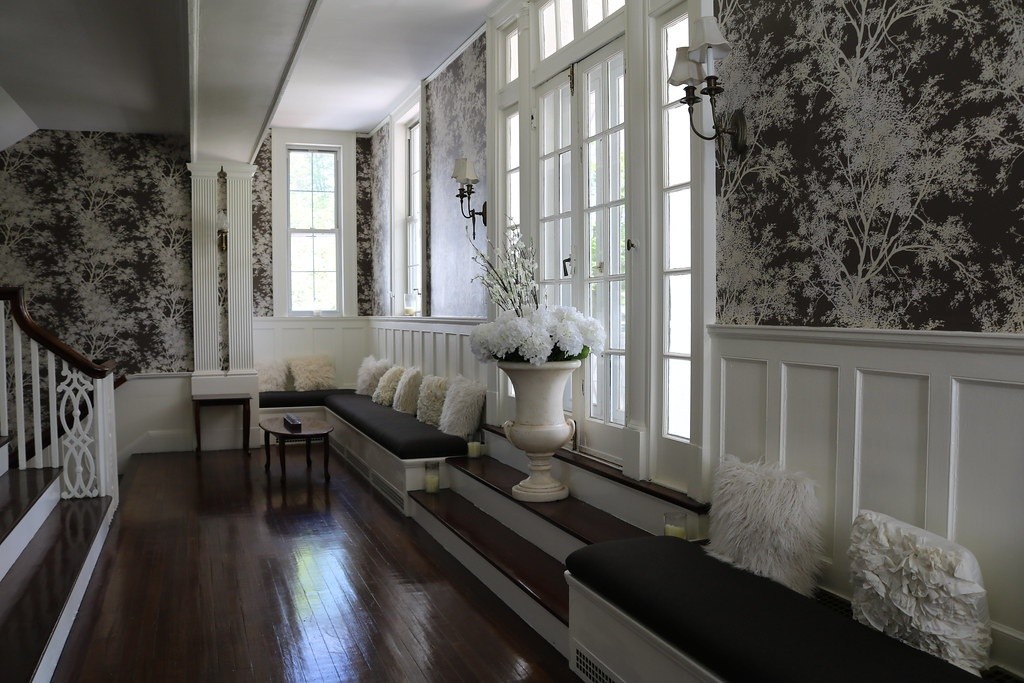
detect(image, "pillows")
[845,508,992,679]
[372,367,406,406]
[255,359,290,392]
[703,454,824,597]
[288,355,336,392]
[354,355,391,397]
[391,367,424,417]
[416,373,450,426]
[436,374,488,440]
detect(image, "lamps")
[450,158,486,240]
[218,210,229,253]
[665,14,738,180]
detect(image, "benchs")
[323,394,472,519]
[564,535,1024,683]
[258,389,357,444]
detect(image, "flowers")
[466,213,608,365]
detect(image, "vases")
[497,359,581,503]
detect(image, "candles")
[425,474,439,494]
[664,523,687,541]
[466,442,483,459]
[405,307,414,317]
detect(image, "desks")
[258,417,335,482]
[191,394,254,456]
